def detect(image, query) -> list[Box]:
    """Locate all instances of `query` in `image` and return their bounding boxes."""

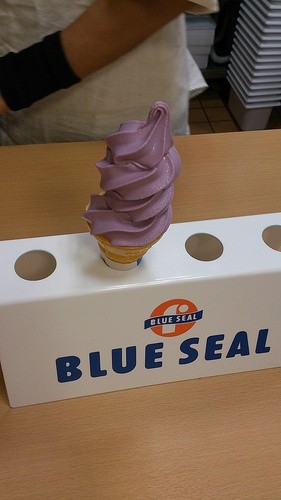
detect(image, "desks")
[1,128,280,498]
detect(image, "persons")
[1,1,220,149]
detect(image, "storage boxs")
[0,212,281,409]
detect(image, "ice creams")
[80,100,182,272]
